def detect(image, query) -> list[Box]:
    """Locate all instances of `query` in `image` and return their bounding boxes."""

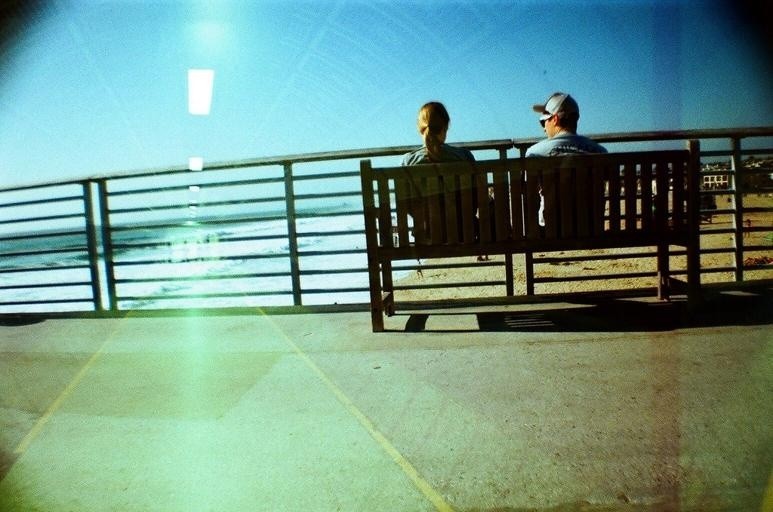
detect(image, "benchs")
[360,139,703,333]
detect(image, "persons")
[522,91,608,239]
[399,101,478,244]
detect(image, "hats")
[533,92,579,121]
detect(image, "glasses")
[538,114,551,127]
